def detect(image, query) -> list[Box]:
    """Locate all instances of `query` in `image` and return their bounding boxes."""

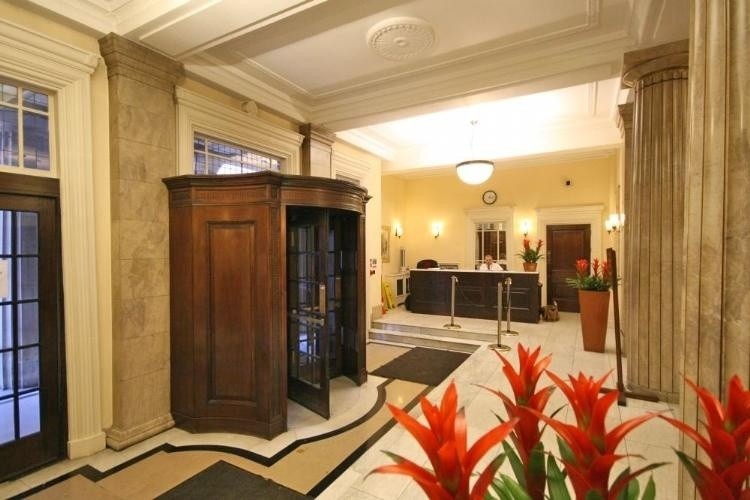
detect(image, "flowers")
[516,238,545,262]
[364,342,749,500]
[565,257,619,291]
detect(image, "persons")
[479,255,504,271]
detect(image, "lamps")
[391,219,446,239]
[606,213,624,233]
[521,218,531,237]
[454,159,494,185]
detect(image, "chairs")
[417,260,437,269]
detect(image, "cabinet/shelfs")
[385,272,410,306]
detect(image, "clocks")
[480,189,497,204]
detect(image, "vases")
[522,261,537,272]
[579,289,611,352]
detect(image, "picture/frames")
[382,228,390,263]
